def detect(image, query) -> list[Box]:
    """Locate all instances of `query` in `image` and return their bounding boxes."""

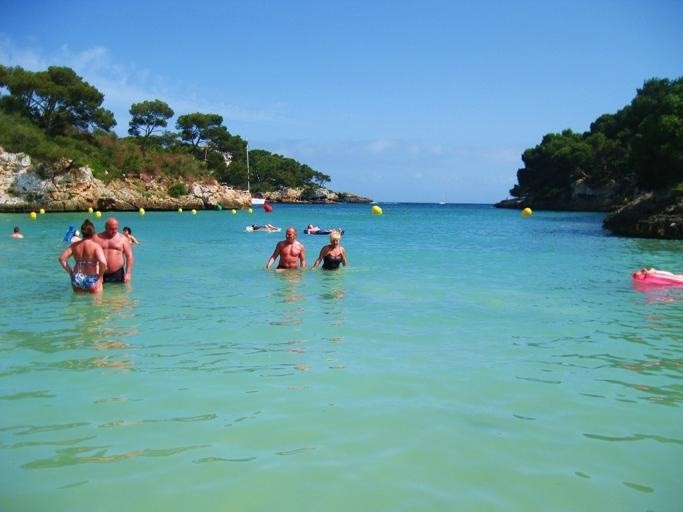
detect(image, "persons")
[640,266,683,280]
[64,224,81,244]
[93,217,133,285]
[122,226,139,245]
[251,223,278,232]
[58,218,107,294]
[308,230,347,273]
[266,227,306,273]
[9,227,23,239]
[306,224,341,236]
